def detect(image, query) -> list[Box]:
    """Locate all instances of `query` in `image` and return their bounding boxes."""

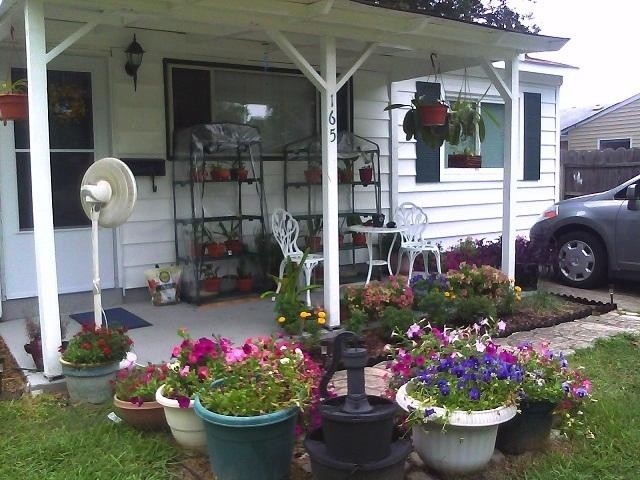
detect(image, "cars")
[528,172,640,289]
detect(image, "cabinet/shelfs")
[173,118,270,305]
[284,128,387,287]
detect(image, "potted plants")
[0,78,29,121]
[385,82,501,149]
[21,318,68,372]
[186,155,372,306]
[448,146,482,168]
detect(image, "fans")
[77,157,139,332]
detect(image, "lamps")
[124,33,146,91]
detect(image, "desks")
[347,224,410,286]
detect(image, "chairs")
[394,203,443,289]
